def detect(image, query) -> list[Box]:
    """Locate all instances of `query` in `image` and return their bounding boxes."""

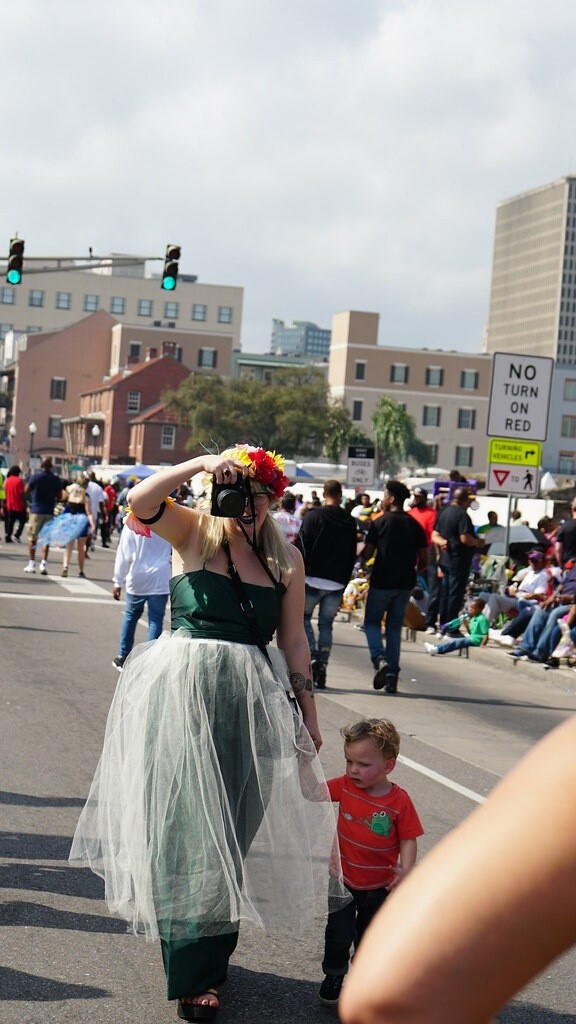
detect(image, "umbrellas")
[484,527,553,546]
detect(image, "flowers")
[202,445,290,506]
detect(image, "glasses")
[253,491,272,505]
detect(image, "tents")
[114,463,157,479]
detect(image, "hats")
[410,488,427,497]
[529,551,543,560]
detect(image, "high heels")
[175,991,219,1022]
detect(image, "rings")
[225,469,230,476]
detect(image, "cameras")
[209,472,248,518]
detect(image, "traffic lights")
[161,243,181,291]
[6,238,25,284]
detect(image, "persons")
[111,514,172,674]
[297,718,426,1001]
[355,478,427,698]
[66,444,324,1024]
[295,479,358,691]
[0,456,203,577]
[336,712,576,1024]
[269,471,576,666]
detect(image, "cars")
[0,453,92,486]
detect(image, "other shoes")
[318,974,344,1003]
[79,572,85,577]
[310,659,327,690]
[112,657,124,671]
[386,683,396,693]
[40,567,47,574]
[487,627,576,670]
[373,667,395,689]
[425,642,435,655]
[0,523,113,559]
[24,565,34,573]
[62,568,67,577]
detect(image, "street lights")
[91,424,101,457]
[29,422,37,458]
[9,426,17,448]
